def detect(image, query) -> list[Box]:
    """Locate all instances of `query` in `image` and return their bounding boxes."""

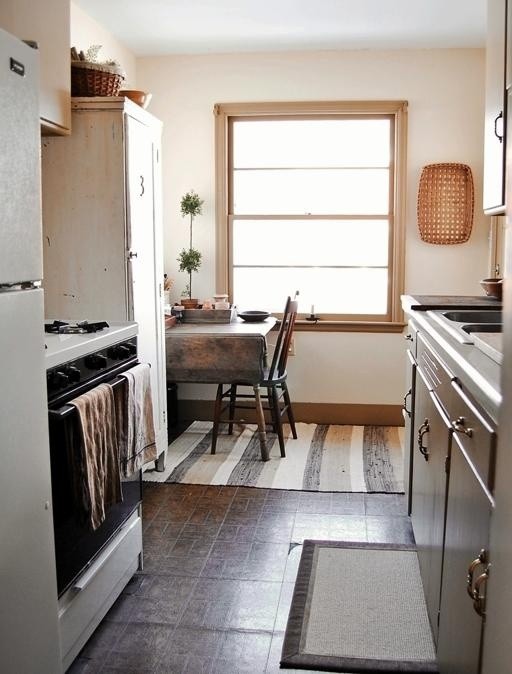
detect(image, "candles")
[310,305,314,314]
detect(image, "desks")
[165,317,278,461]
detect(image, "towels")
[116,362,158,480]
[64,383,125,533]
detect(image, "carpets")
[141,420,406,494]
[279,540,440,674]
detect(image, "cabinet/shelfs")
[401,318,419,517]
[482,0,512,216]
[0,0,72,137]
[41,95,169,472]
[410,333,456,652]
[434,379,512,674]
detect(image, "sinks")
[432,310,503,323]
[447,323,503,336]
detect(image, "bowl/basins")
[214,301,230,311]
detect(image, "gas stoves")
[44,320,139,402]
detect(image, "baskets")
[416,161,476,247]
[71,54,126,97]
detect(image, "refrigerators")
[0,25,61,674]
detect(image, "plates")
[238,311,271,322]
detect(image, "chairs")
[210,295,298,457]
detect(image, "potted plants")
[176,193,204,309]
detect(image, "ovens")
[45,358,152,671]
